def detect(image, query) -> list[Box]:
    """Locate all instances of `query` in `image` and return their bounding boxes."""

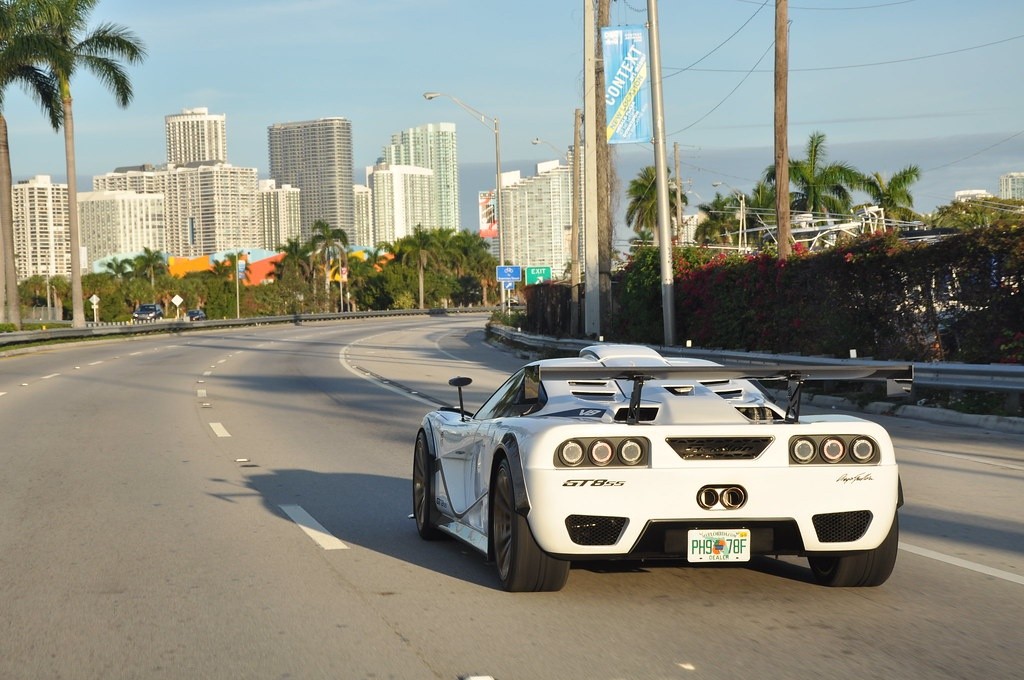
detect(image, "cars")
[132,304,165,322]
[188,308,207,321]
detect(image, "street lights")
[531,137,573,228]
[425,89,505,313]
[710,179,748,255]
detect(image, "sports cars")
[411,339,905,594]
